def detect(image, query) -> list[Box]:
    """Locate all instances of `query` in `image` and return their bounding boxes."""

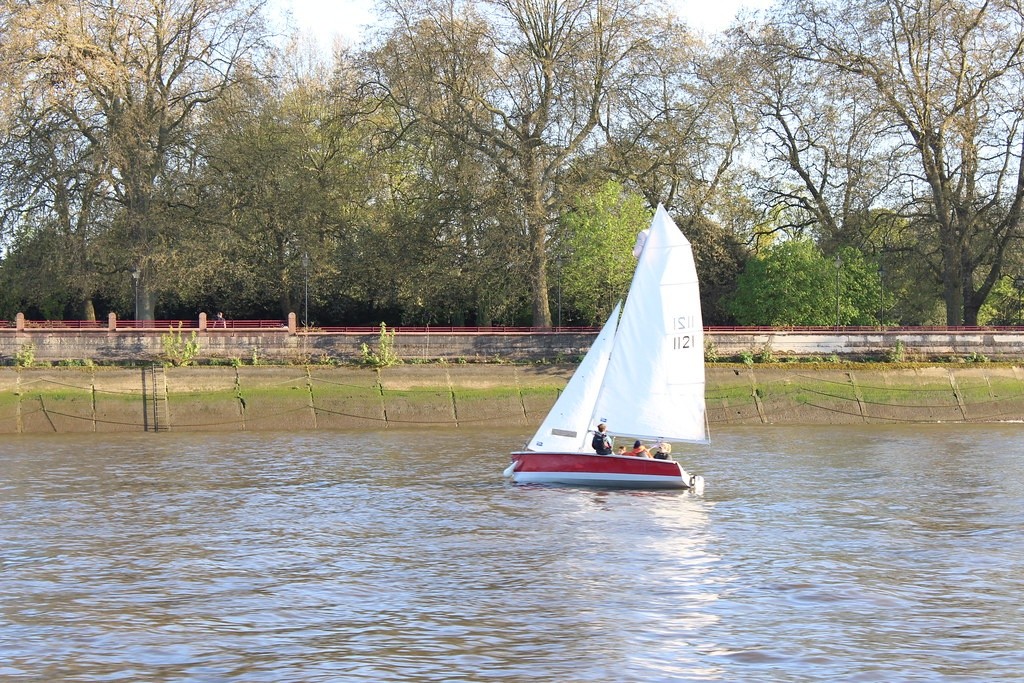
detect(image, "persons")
[592,424,615,455]
[215,312,225,321]
[280,323,288,328]
[618,440,652,458]
[654,442,672,460]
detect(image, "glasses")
[659,447,662,449]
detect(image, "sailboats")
[503,199,714,492]
